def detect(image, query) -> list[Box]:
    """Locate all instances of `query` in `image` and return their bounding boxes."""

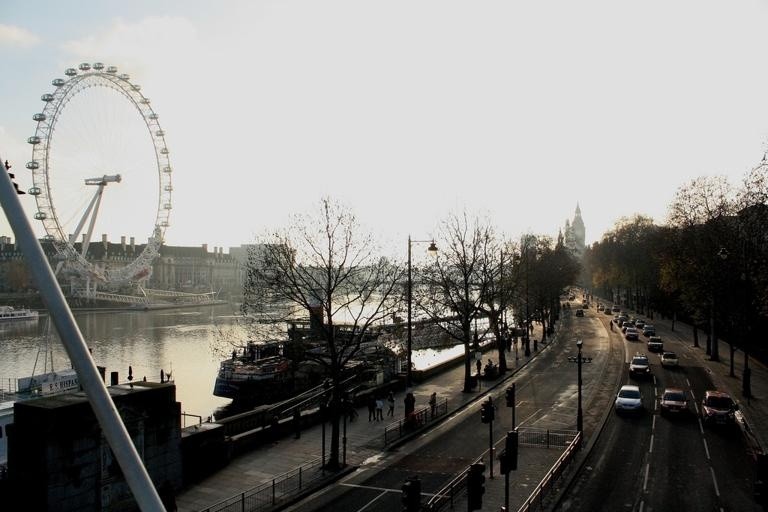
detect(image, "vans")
[701,390,739,427]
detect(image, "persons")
[475,314,540,377]
[609,319,614,331]
[342,389,437,423]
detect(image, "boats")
[211,286,393,401]
[0,306,39,320]
[0,309,106,475]
[0,318,39,333]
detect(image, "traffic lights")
[505,430,517,470]
[469,462,485,511]
[504,386,514,409]
[399,477,422,511]
[481,400,493,424]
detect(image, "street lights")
[405,237,438,413]
[500,250,521,335]
[526,246,542,356]
[717,241,753,400]
[569,338,592,447]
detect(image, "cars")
[599,302,664,354]
[658,387,690,417]
[615,384,645,413]
[567,290,590,318]
[661,352,680,369]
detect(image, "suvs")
[629,352,651,378]
